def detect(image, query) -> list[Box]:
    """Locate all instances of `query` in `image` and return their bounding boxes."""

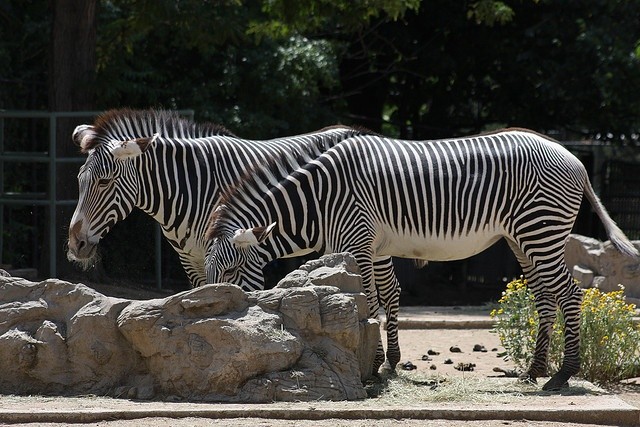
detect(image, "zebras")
[67,108,429,384]
[204,126,640,392]
